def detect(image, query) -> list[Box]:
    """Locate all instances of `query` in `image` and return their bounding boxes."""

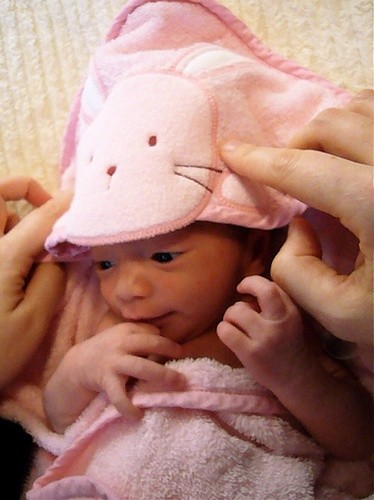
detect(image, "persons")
[44,0,354,500]
[0,85,374,500]
